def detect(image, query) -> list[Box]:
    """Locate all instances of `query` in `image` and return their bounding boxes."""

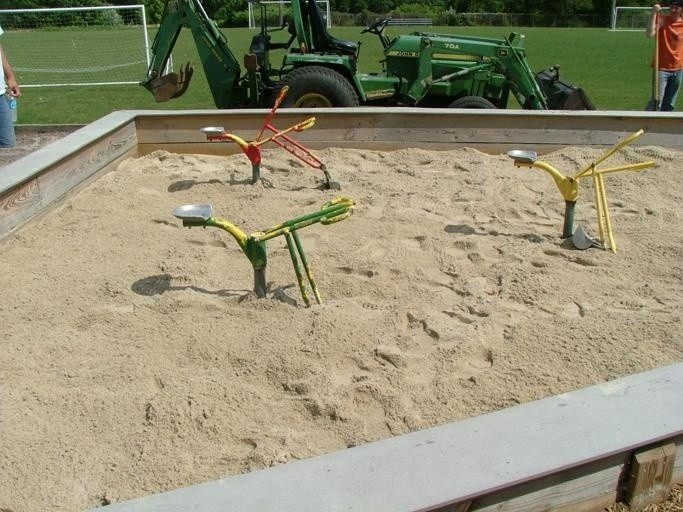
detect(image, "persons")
[0,25,22,148]
[645,0,683,111]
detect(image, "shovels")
[646,13,661,110]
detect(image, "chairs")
[310,0,357,57]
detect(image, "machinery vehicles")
[137,1,599,112]
